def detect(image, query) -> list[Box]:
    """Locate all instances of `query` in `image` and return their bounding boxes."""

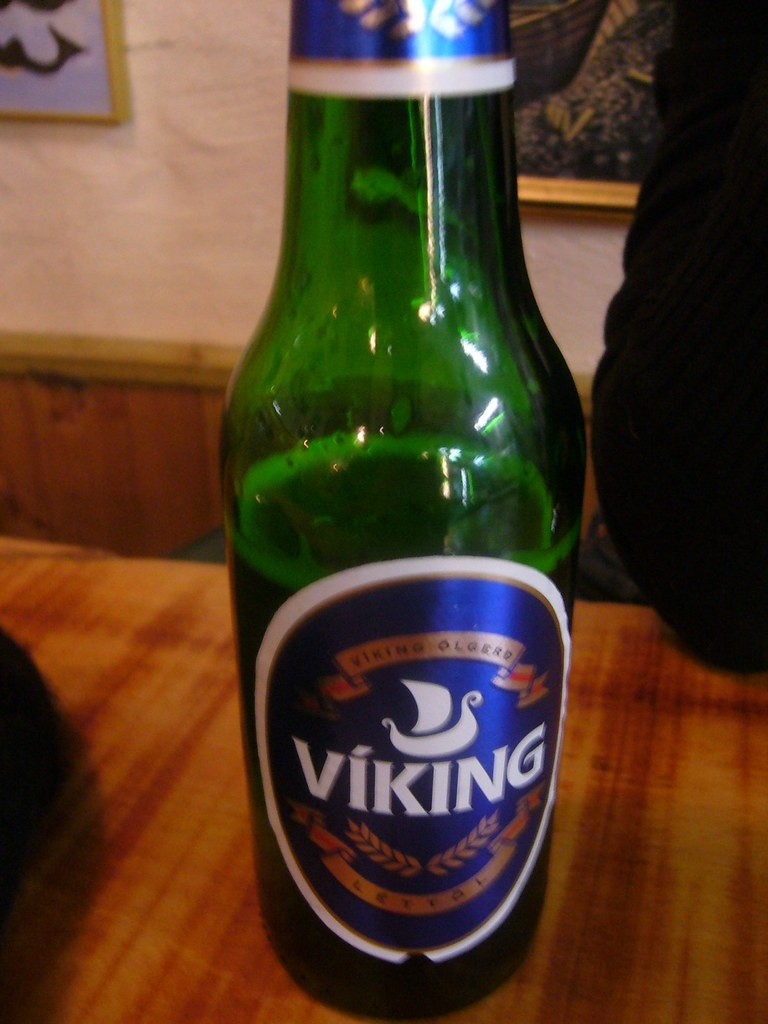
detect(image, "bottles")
[221,1,588,1015]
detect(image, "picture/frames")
[0,0,132,127]
[507,0,674,213]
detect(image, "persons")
[589,0,768,677]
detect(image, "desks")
[1,537,768,1024]
